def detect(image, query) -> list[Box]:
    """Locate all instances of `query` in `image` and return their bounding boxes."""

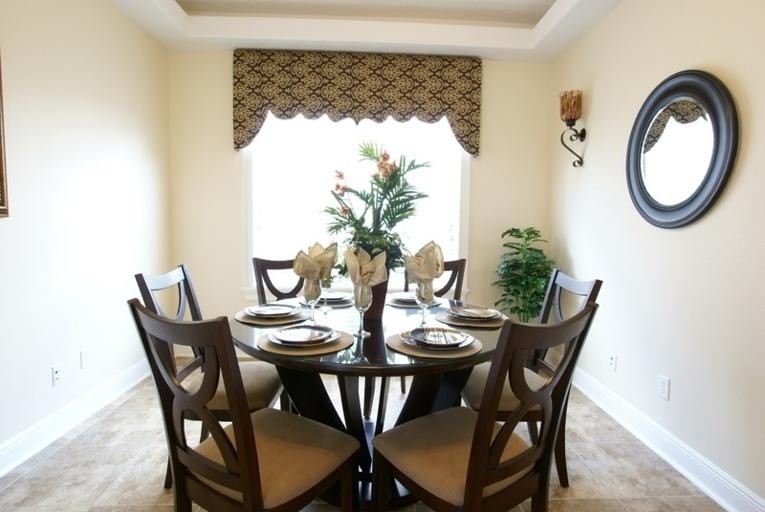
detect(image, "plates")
[247,304,298,315]
[399,330,476,350]
[267,329,342,347]
[448,305,498,317]
[409,327,469,345]
[272,324,334,342]
[243,304,304,319]
[318,293,354,303]
[445,309,503,320]
[392,295,438,303]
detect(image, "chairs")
[463,268,603,488]
[135,265,292,490]
[400,259,466,393]
[128,298,361,512]
[371,302,600,512]
[252,257,306,304]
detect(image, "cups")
[415,280,434,327]
[353,286,372,339]
[303,278,321,324]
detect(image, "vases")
[364,281,390,320]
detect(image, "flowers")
[324,142,430,278]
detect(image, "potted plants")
[491,226,561,368]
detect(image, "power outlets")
[659,377,670,401]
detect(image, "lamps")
[560,91,585,168]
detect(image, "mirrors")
[626,70,738,229]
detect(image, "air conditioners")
[608,353,617,371]
